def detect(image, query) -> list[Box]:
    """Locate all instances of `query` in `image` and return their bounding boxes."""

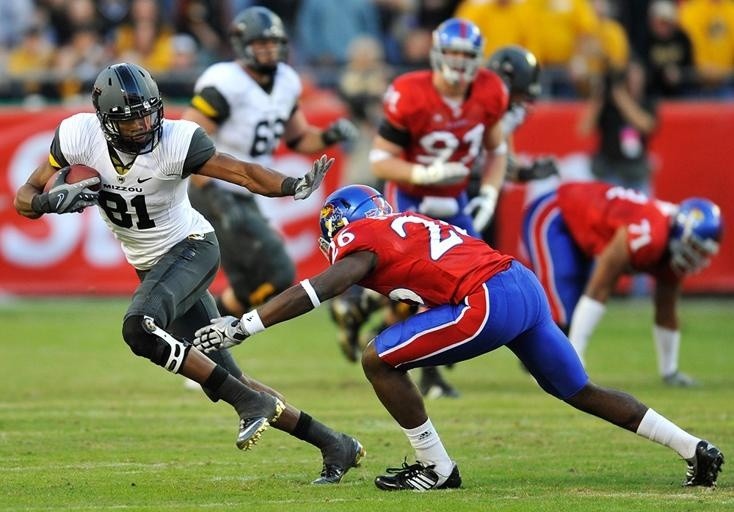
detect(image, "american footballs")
[44,164,101,202]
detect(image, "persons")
[181,4,361,391]
[332,17,511,401]
[191,183,726,492]
[420,47,542,396]
[0,0,734,116]
[11,61,367,485]
[577,59,659,299]
[519,180,725,390]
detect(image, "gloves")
[464,185,499,230]
[281,154,334,201]
[32,167,100,214]
[193,316,249,353]
[663,371,695,387]
[413,162,469,184]
[321,118,355,145]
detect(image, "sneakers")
[419,382,456,398]
[333,298,366,362]
[375,460,461,491]
[682,441,724,486]
[312,438,365,484]
[236,392,285,450]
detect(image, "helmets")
[92,63,163,120]
[669,199,721,272]
[319,184,393,243]
[229,6,287,72]
[487,47,540,101]
[430,18,483,84]
